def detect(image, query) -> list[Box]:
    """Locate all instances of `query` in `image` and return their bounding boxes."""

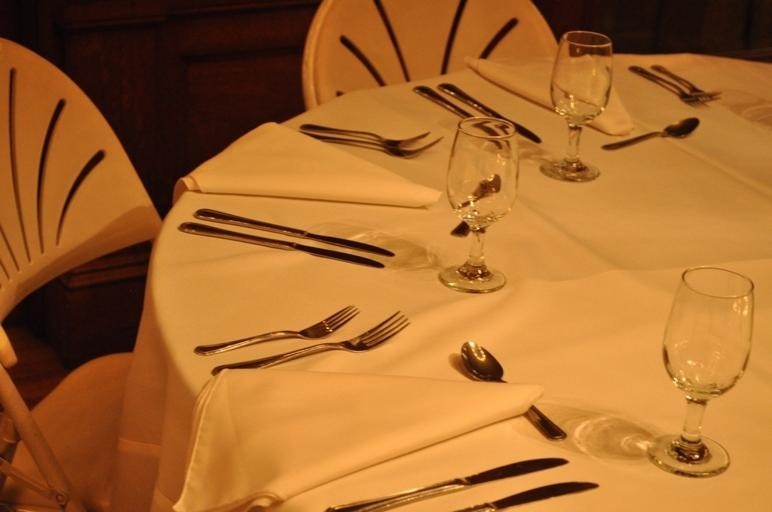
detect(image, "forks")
[194,305,360,356]
[211,311,410,376]
[298,124,444,159]
[628,64,721,103]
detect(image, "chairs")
[302,0,557,111]
[0,37,163,512]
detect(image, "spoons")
[461,341,567,441]
[601,117,700,151]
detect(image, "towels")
[465,53,634,136]
[172,368,544,511]
[172,121,442,209]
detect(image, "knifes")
[437,83,542,144]
[193,208,395,257]
[453,482,599,512]
[413,85,511,150]
[178,222,384,268]
[324,457,569,512]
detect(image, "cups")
[539,31,612,183]
[439,116,519,294]
[647,266,754,479]
